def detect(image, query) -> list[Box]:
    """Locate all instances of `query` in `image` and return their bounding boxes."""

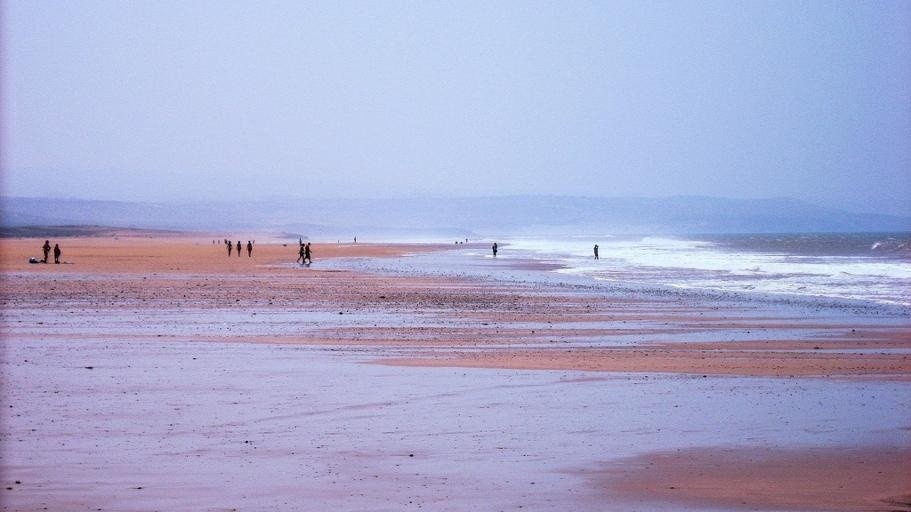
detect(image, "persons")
[247,240,252,257]
[237,241,241,256]
[227,241,232,256]
[54,244,61,263]
[493,243,497,256]
[305,243,313,263]
[594,245,599,259]
[42,240,50,262]
[296,244,305,263]
